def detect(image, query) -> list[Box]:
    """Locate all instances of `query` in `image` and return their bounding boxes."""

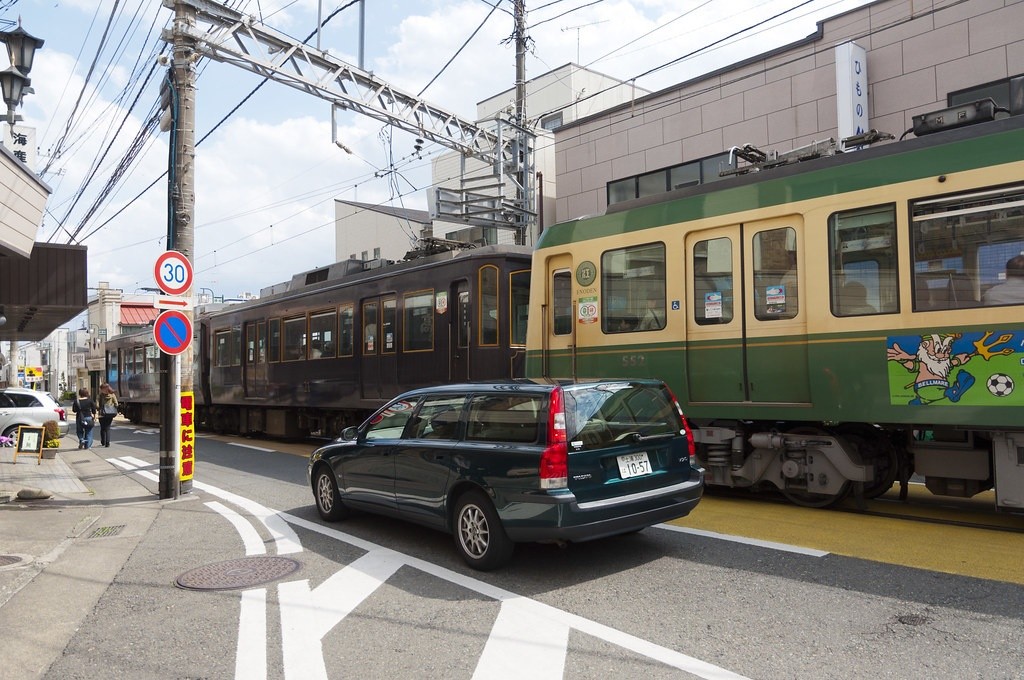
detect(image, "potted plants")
[43,421,61,459]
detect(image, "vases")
[0,446,17,462]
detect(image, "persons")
[777,252,1024,318]
[283,339,334,360]
[95,383,119,447]
[72,388,96,449]
[640,291,666,331]
[619,314,638,331]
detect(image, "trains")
[101,96,1024,514]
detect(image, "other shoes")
[106,442,110,446]
[79,441,85,449]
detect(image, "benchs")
[286,347,304,361]
[915,271,980,307]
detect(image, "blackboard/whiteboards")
[16,425,47,457]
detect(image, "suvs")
[305,377,706,572]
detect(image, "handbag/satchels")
[102,404,116,418]
[80,416,94,428]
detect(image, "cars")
[0,388,69,448]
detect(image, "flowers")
[0,436,12,448]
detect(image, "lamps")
[0,12,46,139]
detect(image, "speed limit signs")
[154,249,194,297]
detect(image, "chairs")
[423,405,457,439]
[479,397,510,438]
[321,341,333,357]
